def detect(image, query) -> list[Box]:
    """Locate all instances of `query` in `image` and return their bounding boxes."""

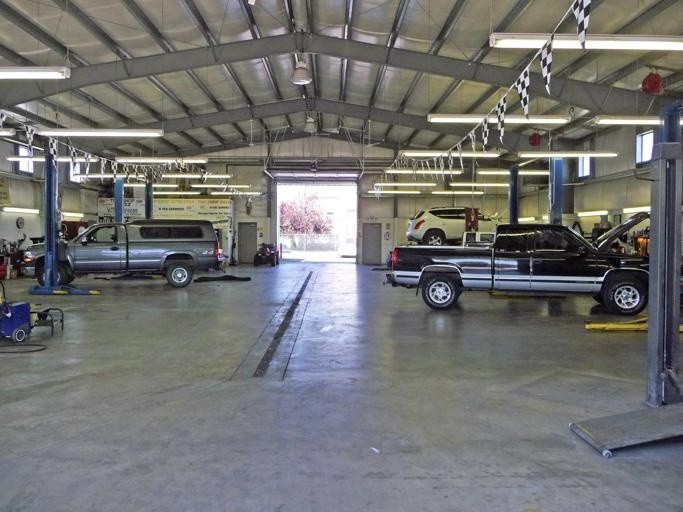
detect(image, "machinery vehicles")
[251,242,280,267]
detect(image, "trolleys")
[0,281,31,342]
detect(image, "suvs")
[404,206,502,246]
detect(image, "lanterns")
[527,133,542,146]
[640,66,664,95]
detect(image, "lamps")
[375,182,438,187]
[517,149,619,159]
[209,191,263,195]
[116,156,209,164]
[161,173,235,179]
[190,183,251,188]
[449,182,510,187]
[33,0,165,137]
[288,53,314,86]
[477,168,551,176]
[73,172,149,178]
[423,0,682,127]
[0,128,17,137]
[6,156,99,162]
[489,0,683,52]
[153,191,202,195]
[302,115,317,136]
[368,190,423,194]
[0,1,72,81]
[123,183,181,188]
[432,190,486,195]
[385,166,464,175]
[395,148,504,159]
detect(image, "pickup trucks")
[19,220,226,289]
[384,211,649,317]
